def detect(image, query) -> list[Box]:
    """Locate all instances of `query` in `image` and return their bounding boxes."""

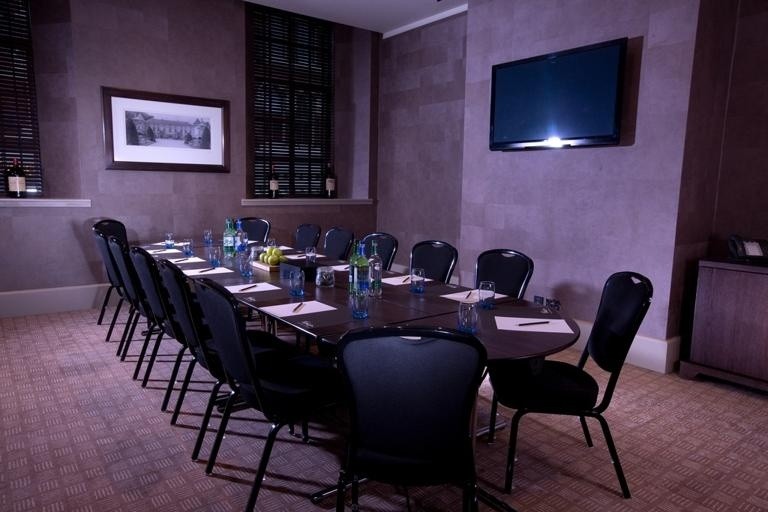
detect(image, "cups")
[208,246,221,267]
[304,245,315,264]
[411,267,425,294]
[239,256,252,276]
[349,287,369,319]
[204,230,213,243]
[290,270,305,298]
[315,267,336,289]
[458,300,477,332]
[479,281,495,308]
[165,232,173,248]
[183,239,194,258]
[267,238,277,246]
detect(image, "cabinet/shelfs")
[677,259,767,396]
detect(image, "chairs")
[322,225,355,260]
[92,218,129,326]
[333,322,491,512]
[106,234,156,382]
[155,257,288,463]
[487,270,654,504]
[191,276,334,512]
[293,223,322,252]
[360,231,399,272]
[474,248,536,301]
[103,225,142,357]
[127,242,189,413]
[408,239,459,285]
[233,215,271,245]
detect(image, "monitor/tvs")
[489,36,629,151]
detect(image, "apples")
[260,253,266,263]
[268,255,279,266]
[279,256,288,262]
[271,248,283,257]
[267,247,275,256]
[264,254,272,264]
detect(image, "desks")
[125,233,580,506]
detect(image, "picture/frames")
[99,85,233,175]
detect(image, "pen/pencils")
[293,302,303,312]
[344,266,349,269]
[466,291,472,299]
[175,259,188,262]
[153,249,166,252]
[402,277,409,282]
[519,321,549,326]
[239,285,256,291]
[297,255,305,257]
[199,268,215,273]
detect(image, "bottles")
[350,240,358,294]
[235,219,244,252]
[354,241,368,297]
[6,158,26,199]
[223,217,237,259]
[325,162,337,198]
[270,165,280,200]
[368,240,383,298]
